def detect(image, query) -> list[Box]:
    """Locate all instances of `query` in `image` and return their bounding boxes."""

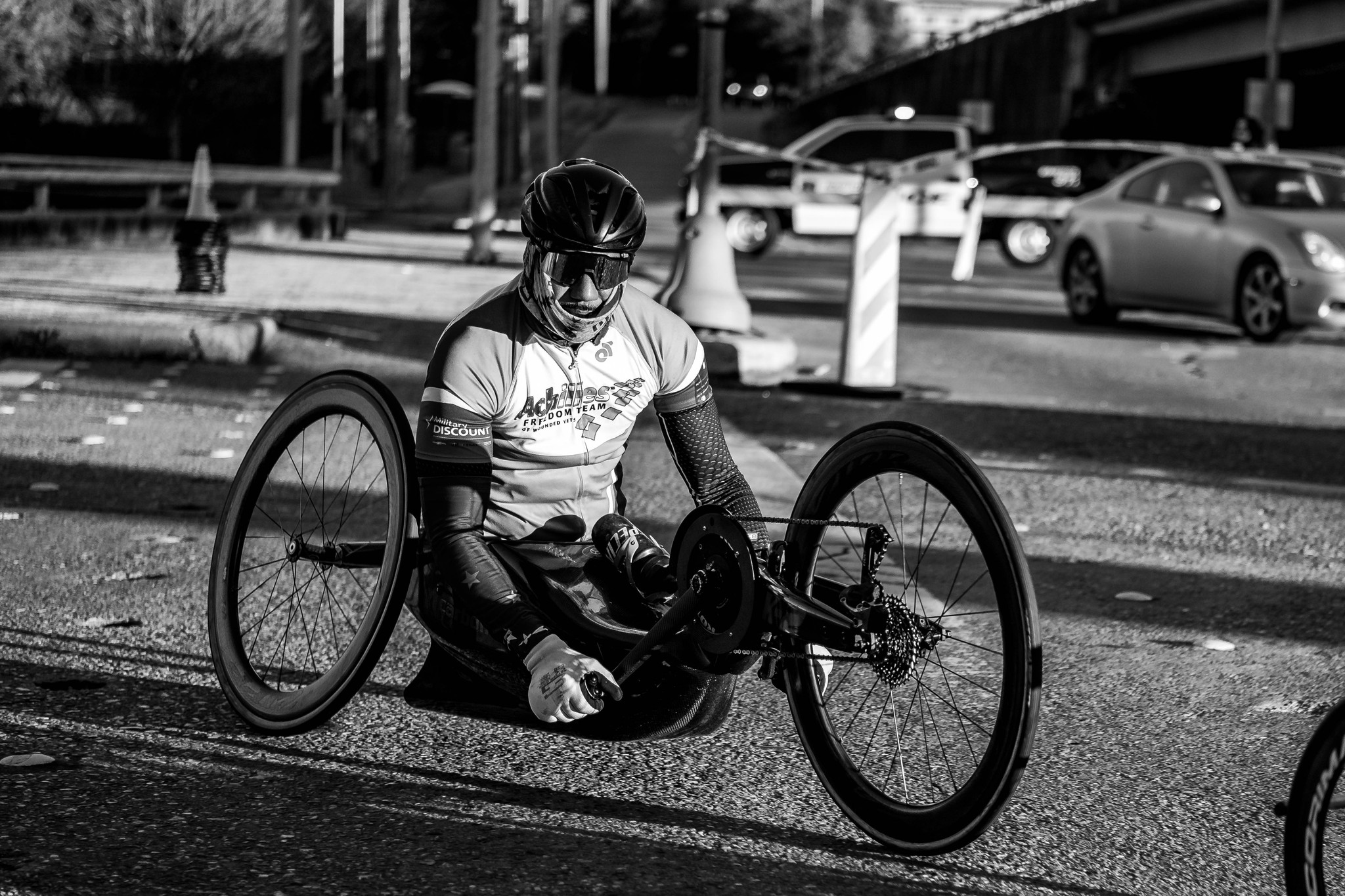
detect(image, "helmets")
[520,157,647,260]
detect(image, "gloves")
[811,645,834,697]
[523,634,623,722]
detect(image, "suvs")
[673,109,980,247]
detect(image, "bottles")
[590,513,679,605]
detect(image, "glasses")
[540,252,629,290]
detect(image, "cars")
[971,135,1204,266]
[1059,147,1345,339]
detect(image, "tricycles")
[207,368,1050,863]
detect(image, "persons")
[412,157,834,722]
